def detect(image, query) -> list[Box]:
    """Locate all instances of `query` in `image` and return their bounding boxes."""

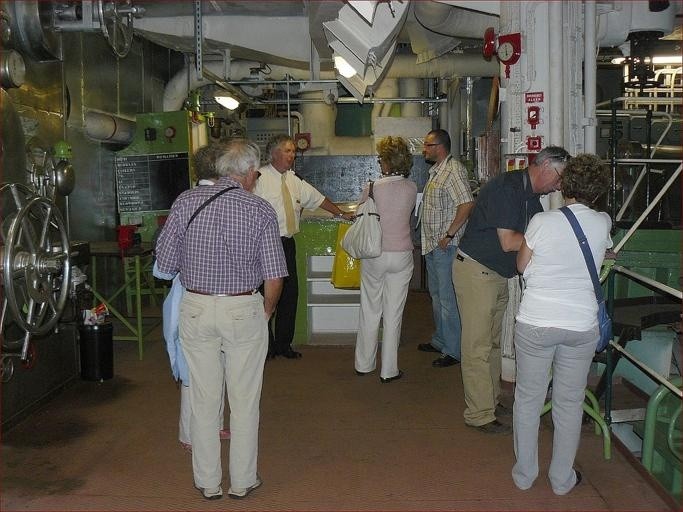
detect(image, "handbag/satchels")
[342,182,382,259]
[596,300,612,353]
[331,223,361,288]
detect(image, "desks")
[83,242,169,358]
[520,251,617,459]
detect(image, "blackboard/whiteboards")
[115,152,191,214]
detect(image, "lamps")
[199,111,216,128]
[215,86,246,111]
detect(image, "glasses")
[376,156,384,165]
[257,171,261,179]
[555,168,563,183]
[424,144,440,148]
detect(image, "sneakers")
[220,430,230,440]
[227,472,262,499]
[575,471,582,486]
[465,420,510,433]
[194,481,223,500]
[494,402,513,417]
[179,442,192,456]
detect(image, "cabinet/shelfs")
[306,252,364,344]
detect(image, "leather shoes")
[432,353,461,367]
[274,346,302,359]
[417,343,441,352]
[380,370,402,383]
[266,342,275,359]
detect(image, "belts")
[186,288,259,296]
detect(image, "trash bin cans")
[77,321,113,381]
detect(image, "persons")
[510,152,613,495]
[154,136,290,499]
[152,140,230,453]
[352,135,418,380]
[254,134,356,358]
[417,129,474,367]
[450,145,574,435]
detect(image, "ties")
[281,175,295,235]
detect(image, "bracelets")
[445,234,453,240]
[338,210,343,218]
